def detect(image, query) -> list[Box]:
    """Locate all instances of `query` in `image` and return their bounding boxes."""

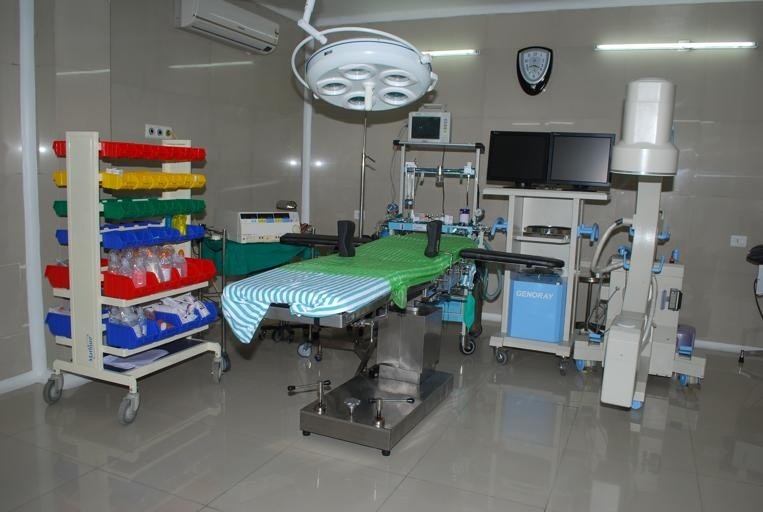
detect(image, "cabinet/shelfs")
[42,130,231,426]
[481,185,612,376]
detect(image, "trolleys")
[198,223,316,372]
[388,139,492,355]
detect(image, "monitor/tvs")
[407,111,451,144]
[547,132,616,193]
[486,131,551,189]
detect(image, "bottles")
[108,246,187,287]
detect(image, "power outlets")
[144,124,172,140]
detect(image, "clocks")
[515,46,553,97]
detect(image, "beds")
[219,234,480,457]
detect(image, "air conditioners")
[172,1,282,57]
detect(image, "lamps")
[292,0,441,114]
